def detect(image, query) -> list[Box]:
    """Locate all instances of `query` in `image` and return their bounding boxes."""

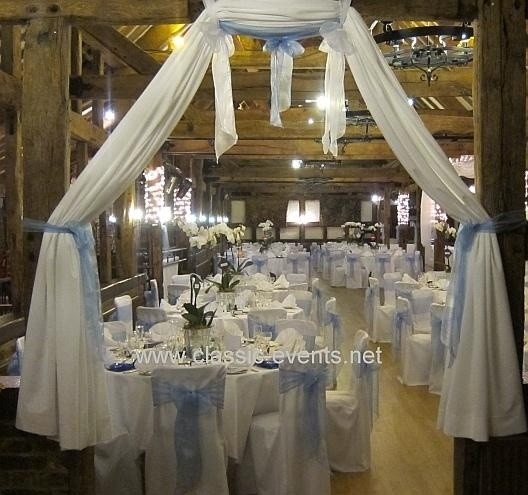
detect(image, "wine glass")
[111,320,284,374]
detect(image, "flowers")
[341,222,384,249]
[178,217,253,294]
[431,220,457,272]
[258,220,276,255]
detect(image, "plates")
[104,358,138,374]
